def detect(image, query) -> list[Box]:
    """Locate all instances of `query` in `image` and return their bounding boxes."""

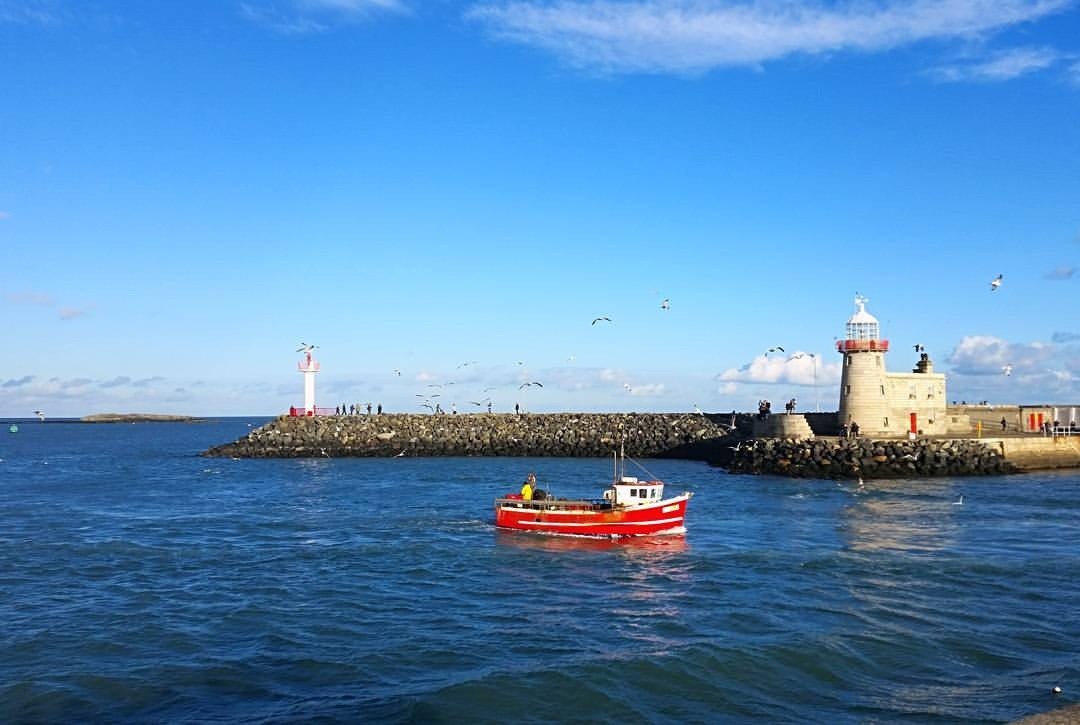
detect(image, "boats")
[488,426,694,542]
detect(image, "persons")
[342,404,346,415]
[1001,417,1005,431]
[452,403,456,415]
[521,481,532,500]
[350,404,360,415]
[367,404,372,414]
[515,403,519,414]
[335,407,340,416]
[377,405,382,415]
[488,402,493,414]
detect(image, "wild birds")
[992,274,1002,291]
[953,496,964,505]
[1002,363,1014,375]
[913,343,928,352]
[295,340,815,451]
[33,410,48,421]
[592,317,612,326]
[660,298,671,311]
